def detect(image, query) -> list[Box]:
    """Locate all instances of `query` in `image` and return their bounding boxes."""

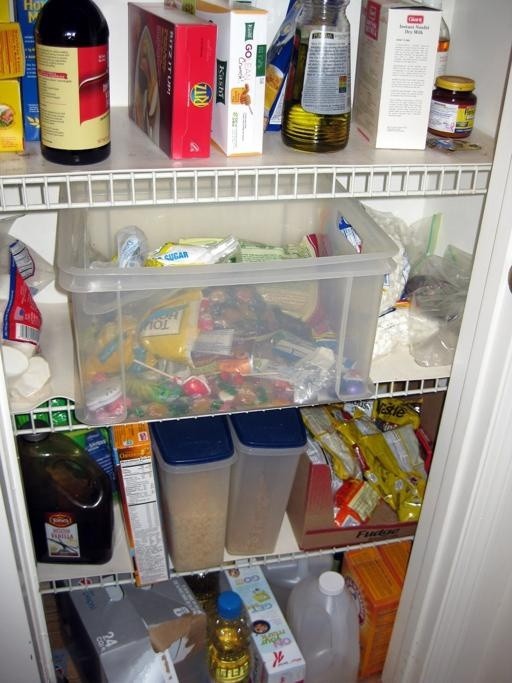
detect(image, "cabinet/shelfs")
[1,1,511,682]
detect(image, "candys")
[77,232,367,425]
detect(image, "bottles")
[33,0,112,166]
[421,0,451,84]
[182,571,252,683]
[151,408,308,573]
[280,1,353,155]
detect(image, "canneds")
[427,75,477,139]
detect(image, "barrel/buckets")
[287,571,360,683]
[14,417,114,566]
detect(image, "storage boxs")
[53,174,400,426]
